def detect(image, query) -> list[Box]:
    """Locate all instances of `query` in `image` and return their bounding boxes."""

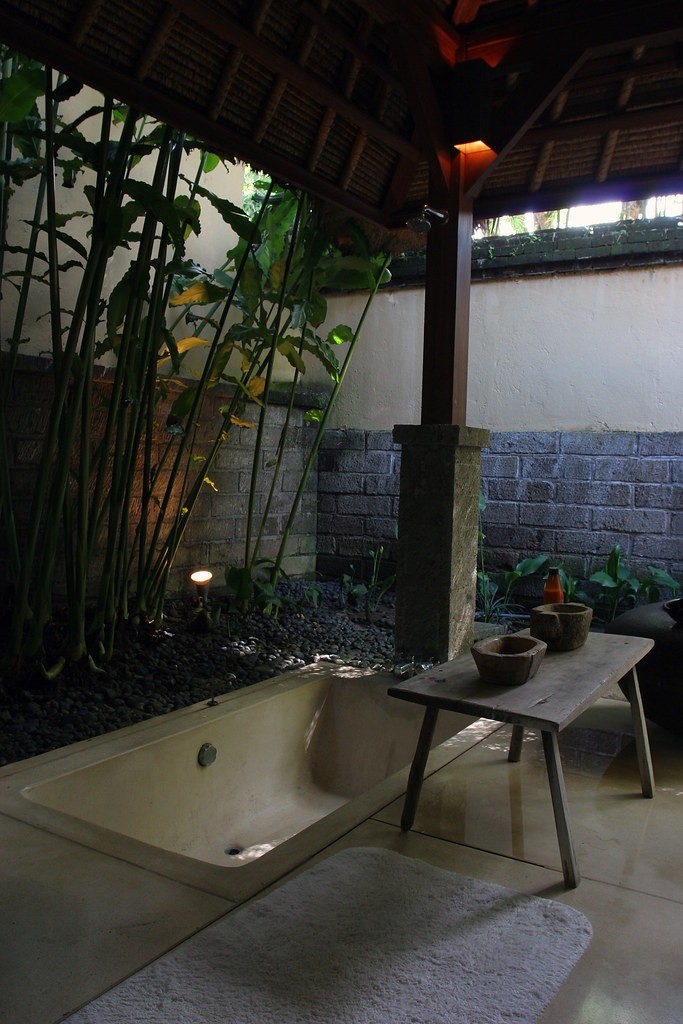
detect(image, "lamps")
[449,57,500,154]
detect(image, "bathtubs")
[0,660,507,905]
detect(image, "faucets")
[206,673,236,706]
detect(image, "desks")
[387,627,655,889]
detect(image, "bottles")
[544,567,563,604]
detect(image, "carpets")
[60,846,595,1024]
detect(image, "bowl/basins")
[530,602,593,651]
[470,634,547,685]
